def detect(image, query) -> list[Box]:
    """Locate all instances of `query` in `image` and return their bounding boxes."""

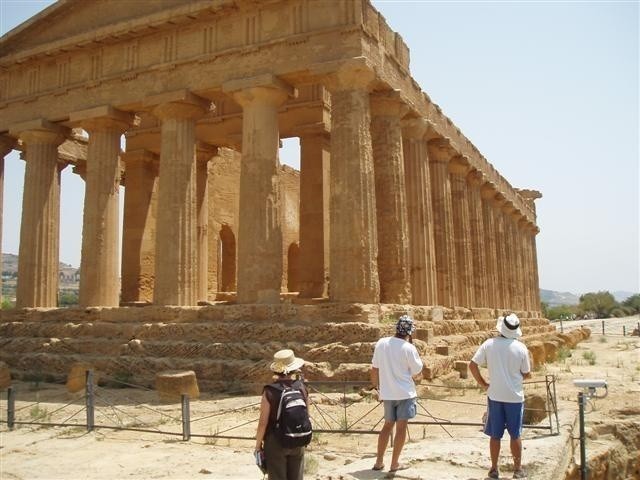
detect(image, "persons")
[468,313,533,478]
[253,348,312,480]
[370,316,425,471]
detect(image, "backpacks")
[263,379,313,449]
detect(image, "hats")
[496,313,523,339]
[270,348,305,373]
[396,315,415,334]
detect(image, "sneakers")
[488,469,499,479]
[513,468,529,479]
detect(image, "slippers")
[388,464,410,473]
[372,465,385,471]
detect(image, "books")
[255,452,265,475]
[258,452,268,474]
